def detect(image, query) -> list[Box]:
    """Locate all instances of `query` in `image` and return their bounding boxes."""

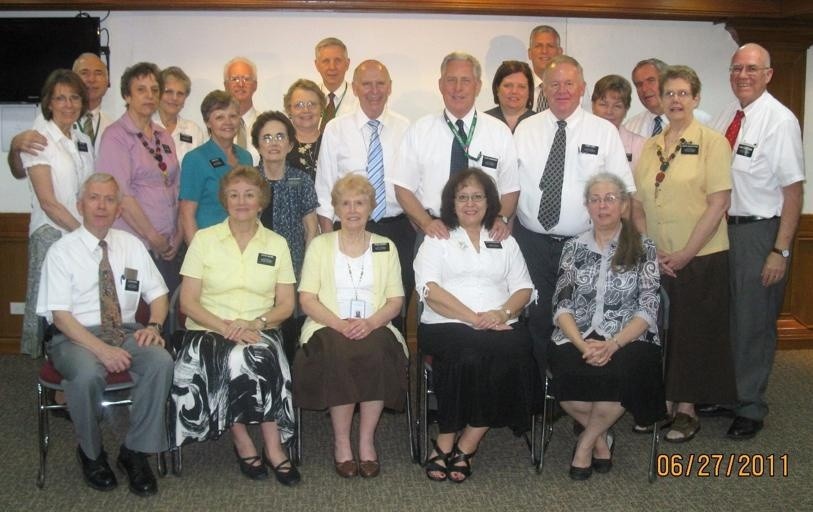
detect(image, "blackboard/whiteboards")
[0,10,740,213]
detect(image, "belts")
[725,213,767,225]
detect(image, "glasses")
[661,89,692,98]
[228,75,252,85]
[257,133,286,143]
[288,101,317,112]
[727,63,770,76]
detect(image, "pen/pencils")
[578,146,580,154]
[120,275,125,284]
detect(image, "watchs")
[772,247,790,258]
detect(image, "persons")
[714,42,807,442]
[635,64,737,443]
[551,172,665,479]
[35,172,173,497]
[169,165,300,486]
[7,23,711,361]
[413,166,544,483]
[293,175,410,478]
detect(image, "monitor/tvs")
[0,17,101,104]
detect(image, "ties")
[724,110,745,149]
[450,119,468,178]
[235,119,247,152]
[650,116,663,139]
[97,240,126,348]
[82,112,95,148]
[536,121,566,232]
[319,92,337,138]
[536,83,549,115]
[364,119,386,224]
